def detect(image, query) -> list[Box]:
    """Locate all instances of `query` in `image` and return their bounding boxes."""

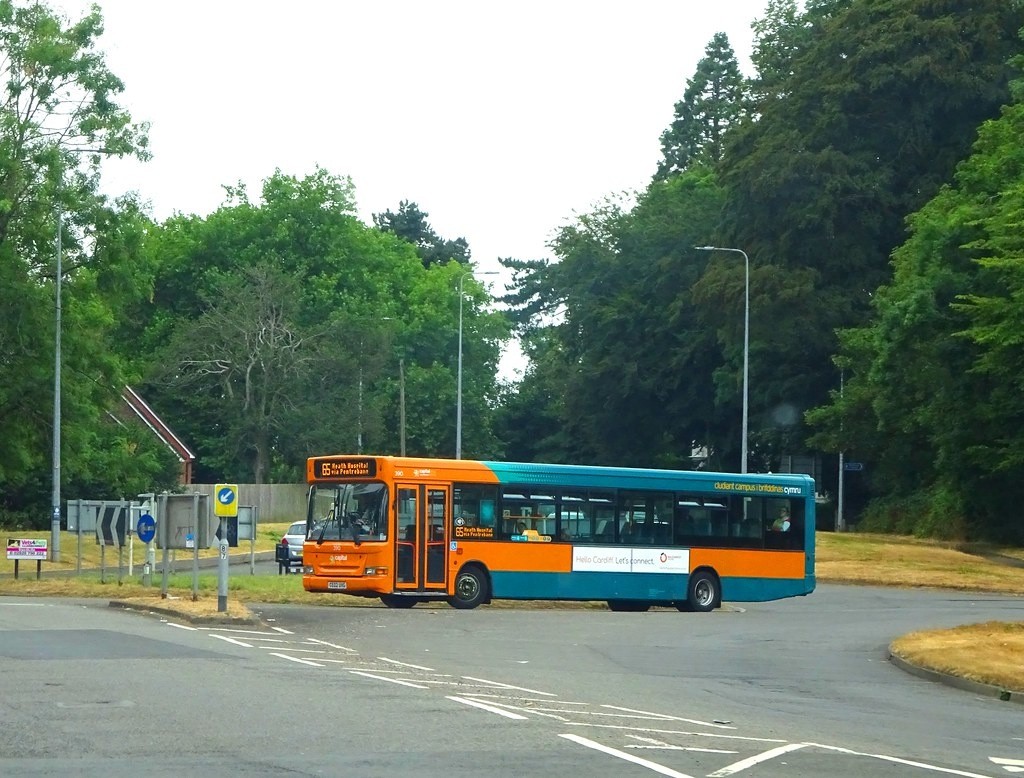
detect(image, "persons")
[768,506,792,533]
[362,490,383,535]
[517,521,529,536]
[561,527,572,541]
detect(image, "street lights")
[694,246,750,523]
[455,271,499,460]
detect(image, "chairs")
[602,521,740,544]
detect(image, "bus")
[303,455,816,613]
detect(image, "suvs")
[281,520,316,563]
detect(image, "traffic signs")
[843,463,862,470]
